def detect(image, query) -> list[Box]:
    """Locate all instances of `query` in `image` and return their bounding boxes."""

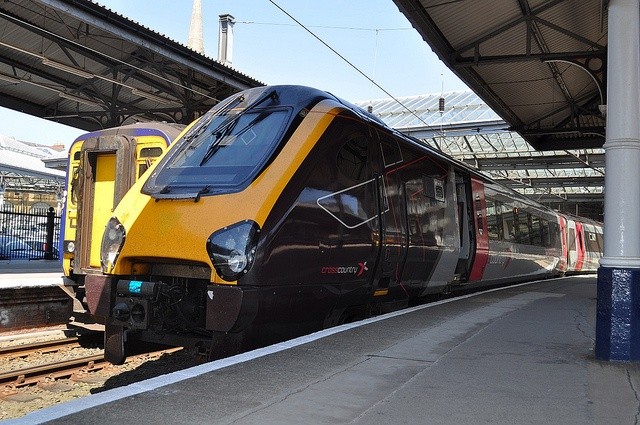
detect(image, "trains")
[85,86,604,365]
[60,121,188,348]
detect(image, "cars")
[4,240,60,259]
[0,235,18,248]
